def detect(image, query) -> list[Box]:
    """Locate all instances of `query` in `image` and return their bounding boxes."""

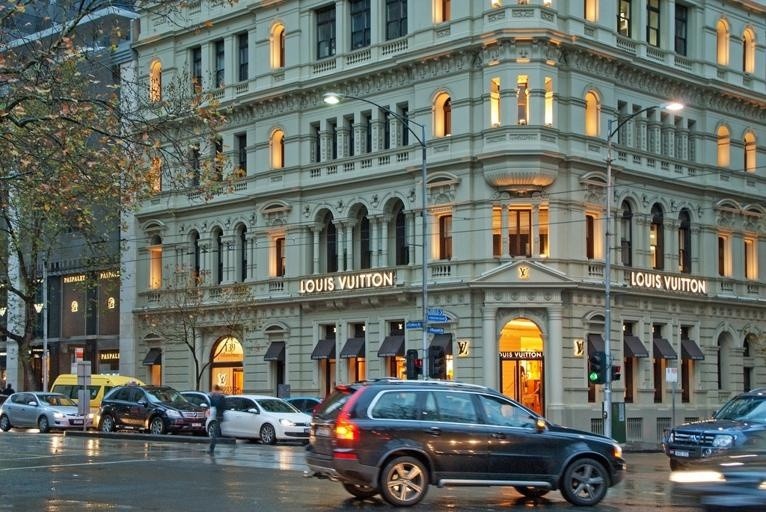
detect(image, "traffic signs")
[408,303,450,340]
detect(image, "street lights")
[322,92,430,376]
[604,100,685,438]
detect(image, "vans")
[48,374,147,428]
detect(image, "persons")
[202,385,224,408]
[2,384,15,401]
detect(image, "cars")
[305,377,628,505]
[0,392,94,434]
[665,390,766,507]
[204,395,312,445]
[179,390,213,408]
[285,397,323,416]
[95,383,207,435]
